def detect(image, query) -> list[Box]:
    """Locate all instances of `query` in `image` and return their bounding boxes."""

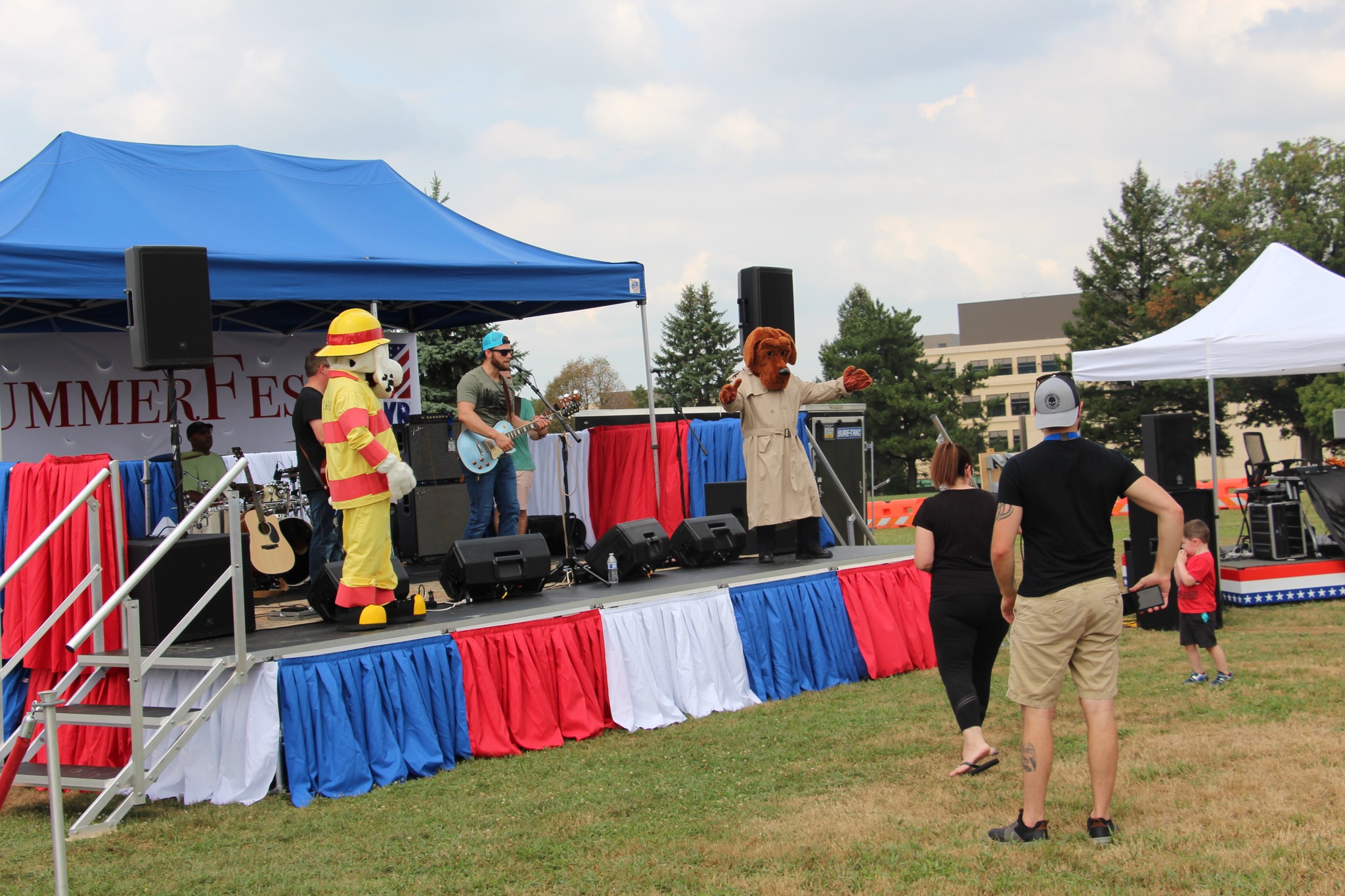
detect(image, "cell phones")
[1123,584,1164,616]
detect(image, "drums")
[185,504,229,534]
[217,503,255,516]
[260,481,294,516]
[272,517,314,586]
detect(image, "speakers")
[668,514,749,567]
[438,532,551,600]
[587,517,668,583]
[413,485,475,555]
[390,423,411,465]
[407,421,467,483]
[127,534,255,647]
[1139,412,1198,490]
[705,481,800,555]
[739,266,796,353]
[307,554,410,622]
[1128,489,1222,632]
[389,489,417,560]
[124,244,212,370]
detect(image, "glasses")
[1035,371,1076,397]
[490,349,514,357]
[198,428,213,434]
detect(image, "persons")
[985,376,1183,844]
[913,443,1025,776]
[182,421,229,509]
[491,369,552,536]
[1173,518,1233,686]
[291,349,347,585]
[454,332,552,540]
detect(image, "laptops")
[1322,409,1345,447]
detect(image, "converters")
[423,601,437,608]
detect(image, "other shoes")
[334,601,387,631]
[381,593,427,621]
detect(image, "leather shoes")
[795,547,833,559]
[759,548,774,562]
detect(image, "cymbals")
[280,467,299,473]
[148,452,204,463]
[271,473,299,478]
[229,483,264,499]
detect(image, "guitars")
[456,400,580,474]
[227,446,295,574]
[530,390,579,422]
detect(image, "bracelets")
[537,432,547,438]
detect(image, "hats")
[1035,376,1080,429]
[476,332,511,361]
[186,422,214,437]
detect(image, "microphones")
[447,416,453,438]
[509,360,532,374]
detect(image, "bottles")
[607,553,619,584]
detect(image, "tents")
[0,130,666,529]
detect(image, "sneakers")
[988,808,1050,845]
[1182,671,1209,685]
[1211,671,1233,687]
[1086,816,1115,844]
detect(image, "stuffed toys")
[315,308,429,630]
[715,324,873,561]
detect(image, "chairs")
[1240,430,1306,544]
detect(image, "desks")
[1234,487,1269,552]
[1264,469,1345,559]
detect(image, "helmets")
[314,308,391,357]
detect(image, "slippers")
[954,751,1000,775]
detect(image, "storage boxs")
[1250,499,1308,559]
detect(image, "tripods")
[520,374,610,586]
